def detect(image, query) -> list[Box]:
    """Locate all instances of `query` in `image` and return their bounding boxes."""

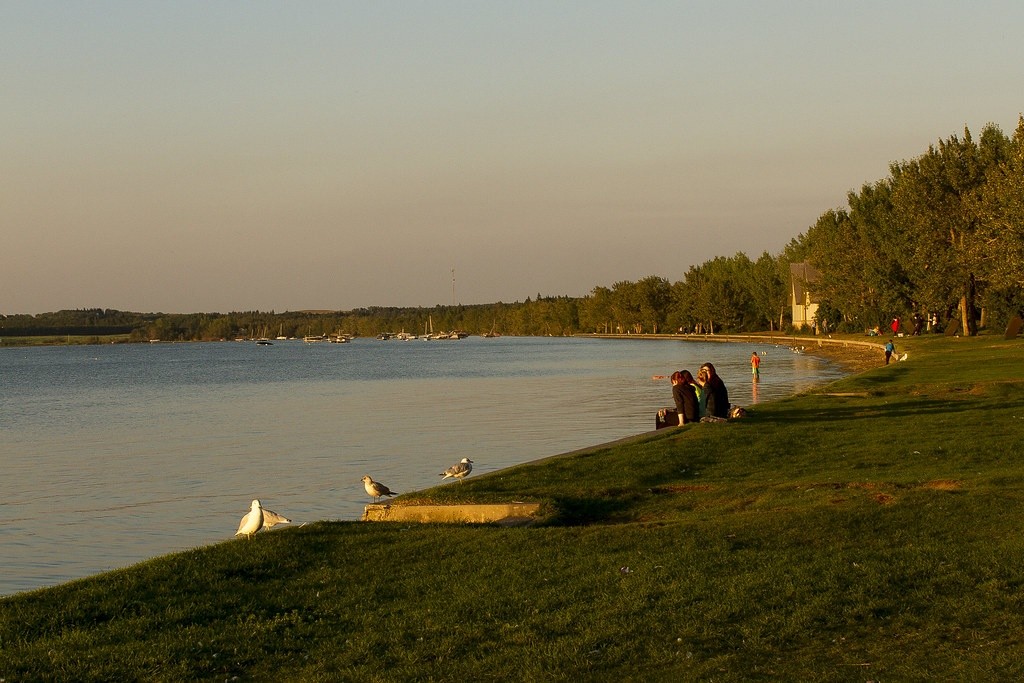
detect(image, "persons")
[656,370,701,431]
[812,318,817,335]
[867,311,938,336]
[884,339,896,364]
[696,369,715,419]
[751,351,760,378]
[678,368,707,419]
[822,317,828,334]
[699,361,730,420]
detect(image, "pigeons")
[438,458,474,485]
[360,476,399,503]
[899,354,908,361]
[249,506,291,530]
[234,500,263,540]
